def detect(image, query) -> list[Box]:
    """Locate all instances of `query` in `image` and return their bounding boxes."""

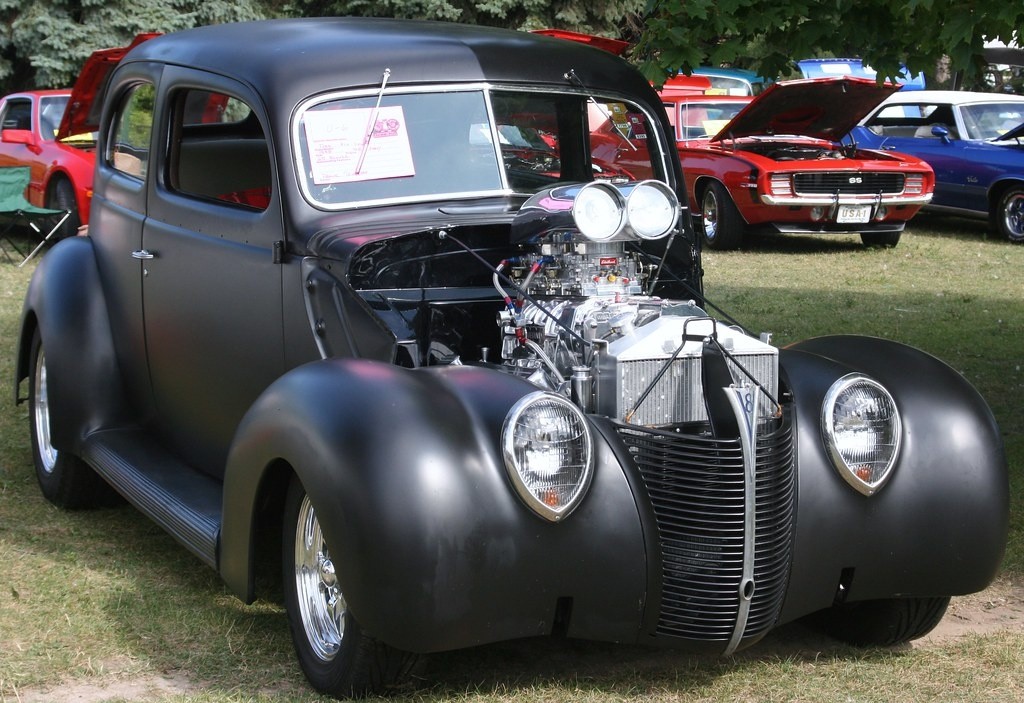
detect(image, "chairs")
[0,166,72,268]
[916,124,952,139]
[177,140,272,197]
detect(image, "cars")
[14,15,1010,698]
[473,28,638,186]
[663,63,780,122]
[796,58,1024,243]
[543,75,938,248]
[952,46,1024,96]
[0,30,270,241]
